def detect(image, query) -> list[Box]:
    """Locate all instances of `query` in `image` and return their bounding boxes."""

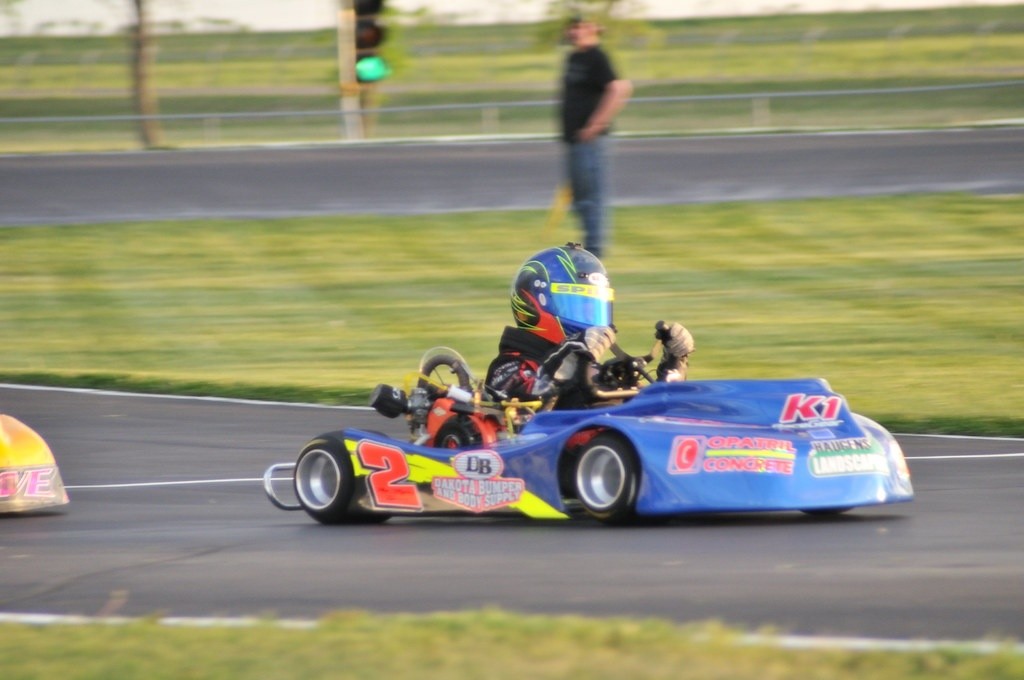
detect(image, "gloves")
[543,326,616,382]
[656,320,694,383]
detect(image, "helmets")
[511,241,615,346]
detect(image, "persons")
[559,15,630,258]
[484,242,693,410]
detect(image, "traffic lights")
[351,0,389,85]
